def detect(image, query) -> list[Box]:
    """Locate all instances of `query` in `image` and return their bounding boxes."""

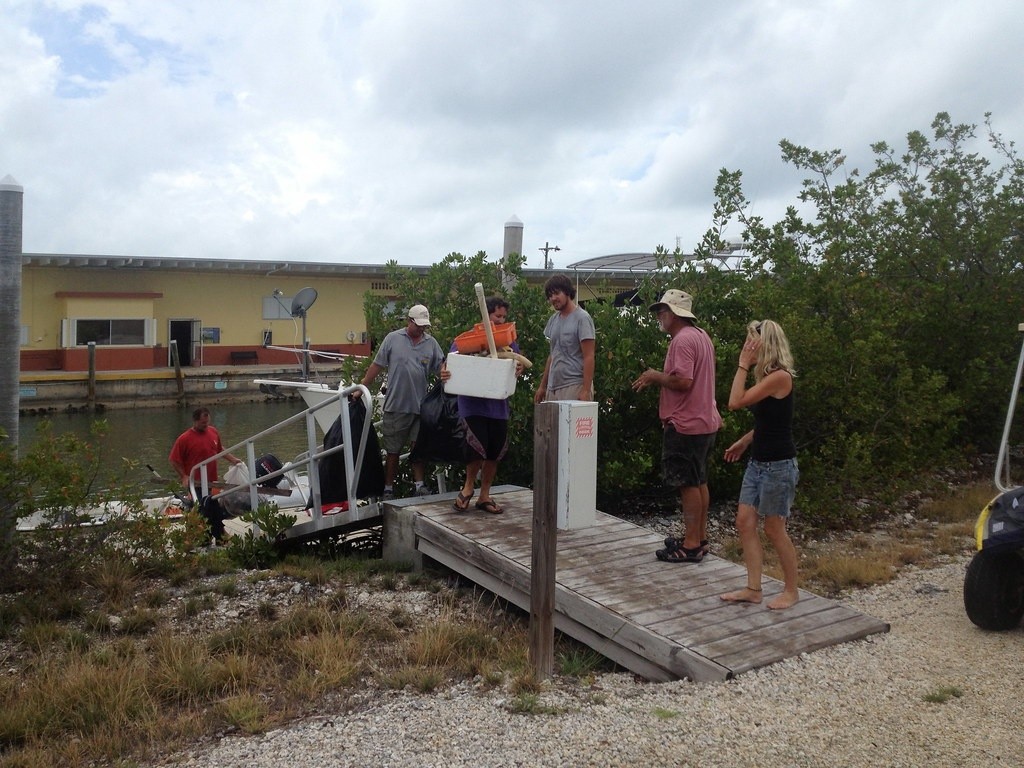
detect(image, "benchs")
[316,349,342,363]
[231,351,259,366]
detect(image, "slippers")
[452,489,474,512]
[475,499,503,514]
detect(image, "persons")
[631,290,725,562]
[353,297,524,514]
[533,274,596,402]
[169,407,241,548]
[720,319,799,610]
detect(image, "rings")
[639,382,641,385]
[751,346,755,350]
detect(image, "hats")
[648,289,698,323]
[408,305,431,327]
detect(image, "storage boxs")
[454,321,517,354]
[443,351,517,399]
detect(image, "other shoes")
[415,486,430,495]
[383,489,393,501]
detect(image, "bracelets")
[737,365,751,373]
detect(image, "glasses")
[755,322,762,335]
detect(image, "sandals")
[656,542,703,562]
[664,537,710,555]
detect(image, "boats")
[15,475,310,532]
[253,244,750,435]
